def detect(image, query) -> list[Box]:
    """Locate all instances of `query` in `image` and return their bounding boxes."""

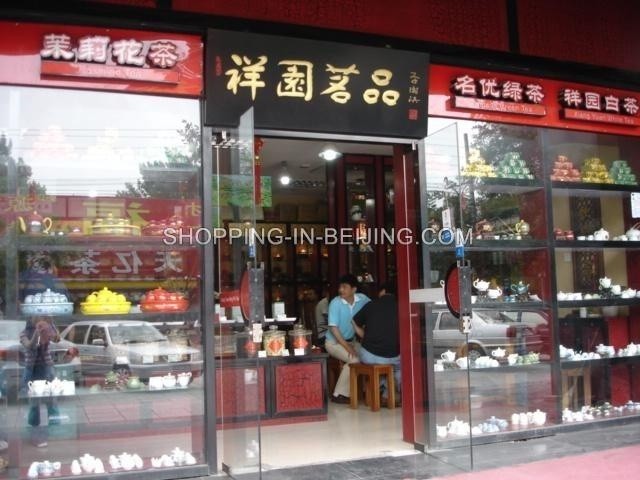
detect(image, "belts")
[344,338,356,343]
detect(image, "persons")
[20,316,60,448]
[351,282,401,407]
[315,291,332,353]
[325,273,372,403]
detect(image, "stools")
[347,362,396,411]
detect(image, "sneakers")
[380,398,402,408]
[47,424,66,435]
[29,434,47,447]
[332,395,352,405]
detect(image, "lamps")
[317,141,342,161]
[277,160,292,186]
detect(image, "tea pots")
[473,278,491,292]
[626,227,640,241]
[441,349,457,362]
[104,371,119,384]
[491,346,506,359]
[456,356,470,370]
[27,380,47,398]
[91,286,117,300]
[599,276,612,288]
[150,286,170,300]
[594,227,609,241]
[42,288,59,303]
[510,280,531,294]
[515,219,530,235]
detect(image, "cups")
[586,401,614,416]
[509,295,519,302]
[54,293,60,302]
[474,355,501,368]
[24,294,33,304]
[513,233,521,240]
[556,290,583,301]
[163,372,175,388]
[584,294,592,299]
[150,446,197,468]
[500,234,508,240]
[561,407,595,423]
[176,371,193,389]
[559,342,615,361]
[91,383,104,393]
[474,356,500,368]
[32,292,41,303]
[108,297,116,303]
[157,293,167,300]
[577,236,585,240]
[510,408,547,426]
[69,453,106,476]
[60,295,68,303]
[587,234,593,240]
[149,376,164,391]
[489,287,503,299]
[436,415,483,438]
[508,351,540,366]
[146,290,154,301]
[613,227,628,241]
[593,294,601,300]
[503,296,511,303]
[27,460,61,479]
[479,415,508,433]
[433,360,444,371]
[166,293,177,300]
[176,292,184,300]
[62,380,76,396]
[47,376,68,396]
[86,295,97,303]
[124,376,140,389]
[615,400,639,414]
[109,452,144,471]
[118,293,125,303]
[618,341,640,357]
[508,233,515,240]
[471,296,477,304]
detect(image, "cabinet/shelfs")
[14,230,206,480]
[324,152,395,301]
[224,219,330,323]
[423,125,640,450]
[209,352,331,430]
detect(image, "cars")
[433,308,536,360]
[500,309,551,337]
[59,320,203,388]
[1,319,85,402]
